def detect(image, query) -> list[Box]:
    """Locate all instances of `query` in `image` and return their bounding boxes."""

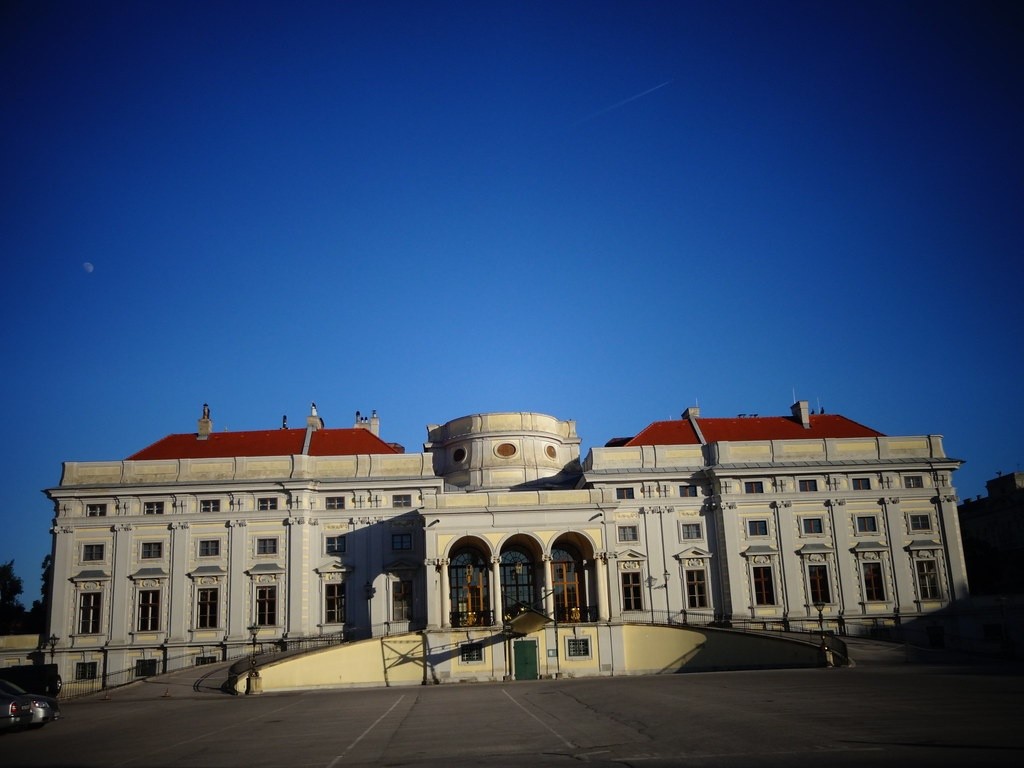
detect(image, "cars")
[0,689,33,729]
[0,680,62,726]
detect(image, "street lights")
[812,601,834,669]
[50,635,60,664]
[247,623,263,695]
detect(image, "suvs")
[0,667,62,697]
